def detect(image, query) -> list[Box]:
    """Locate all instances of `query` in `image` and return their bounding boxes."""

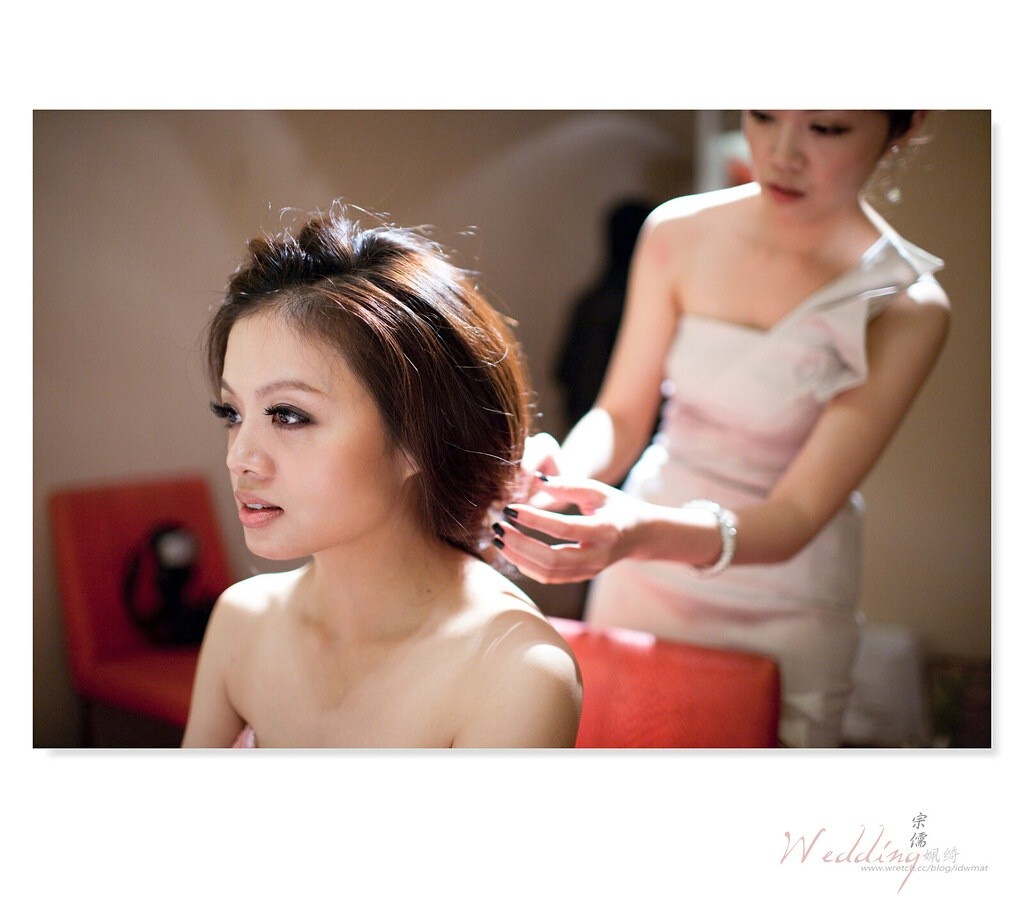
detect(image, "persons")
[470,109,954,750]
[172,205,587,750]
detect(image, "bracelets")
[680,500,736,575]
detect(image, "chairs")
[542,616,781,749]
[48,470,233,748]
